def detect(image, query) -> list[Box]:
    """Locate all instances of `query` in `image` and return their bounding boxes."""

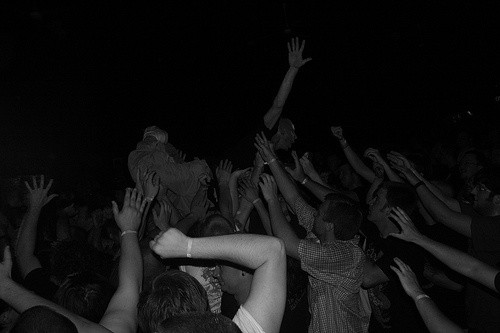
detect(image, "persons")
[0,36,500,333]
